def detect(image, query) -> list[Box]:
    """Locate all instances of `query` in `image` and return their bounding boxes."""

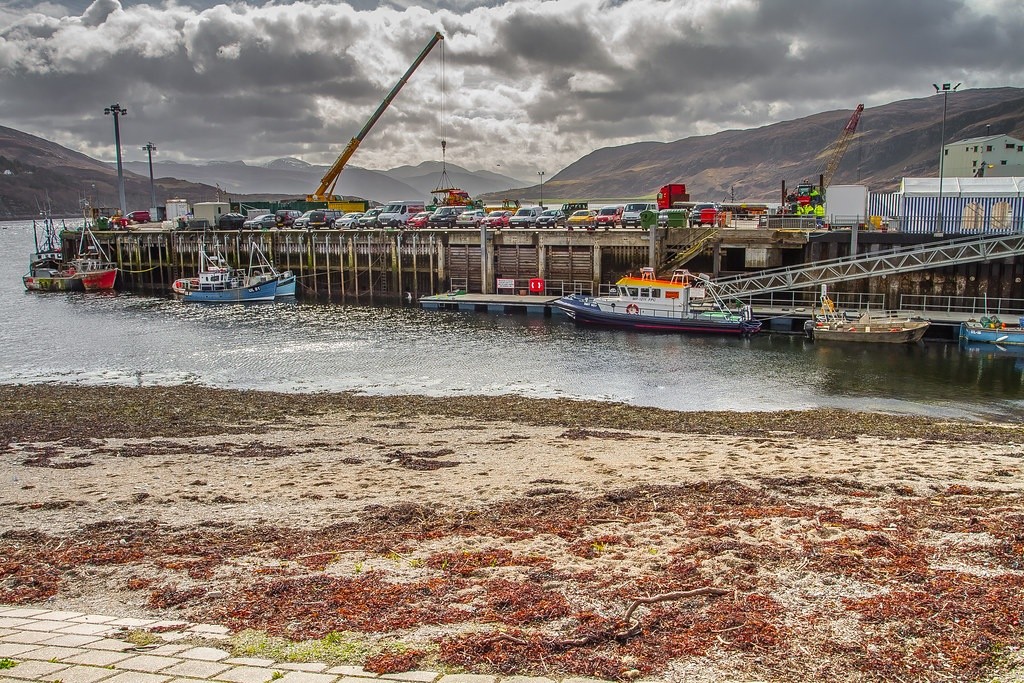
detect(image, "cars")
[481,210,512,227]
[568,210,598,228]
[335,212,366,229]
[456,211,488,229]
[406,211,434,228]
[293,209,316,229]
[535,210,566,228]
[243,214,276,229]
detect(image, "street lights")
[933,82,962,232]
[140,144,157,207]
[104,103,128,217]
[538,172,545,205]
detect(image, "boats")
[172,234,298,303]
[802,292,933,345]
[959,316,1024,345]
[553,267,762,335]
[22,188,119,290]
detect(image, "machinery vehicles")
[305,31,447,202]
[780,103,864,215]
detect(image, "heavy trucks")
[656,183,769,219]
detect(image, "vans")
[126,211,149,223]
[376,200,425,227]
[508,206,543,229]
[358,208,384,229]
[428,206,469,229]
[595,203,658,229]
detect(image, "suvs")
[275,210,304,228]
[688,202,723,227]
[309,209,347,229]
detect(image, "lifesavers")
[849,328,856,331]
[890,329,900,331]
[626,304,640,316]
[531,281,541,289]
